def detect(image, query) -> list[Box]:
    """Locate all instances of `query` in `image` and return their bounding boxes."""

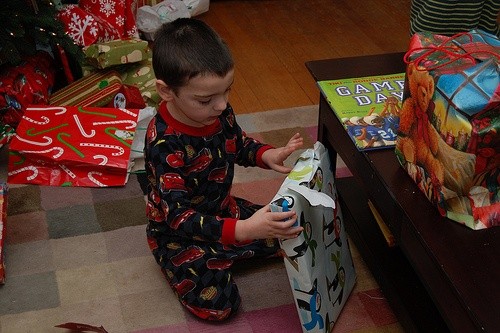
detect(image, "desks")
[304,51,500,333]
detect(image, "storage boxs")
[106,83,147,108]
[85,37,149,68]
[49,67,122,106]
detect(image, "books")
[316,72,408,152]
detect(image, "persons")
[341,96,402,148]
[142,16,303,327]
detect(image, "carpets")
[2,103,409,332]
